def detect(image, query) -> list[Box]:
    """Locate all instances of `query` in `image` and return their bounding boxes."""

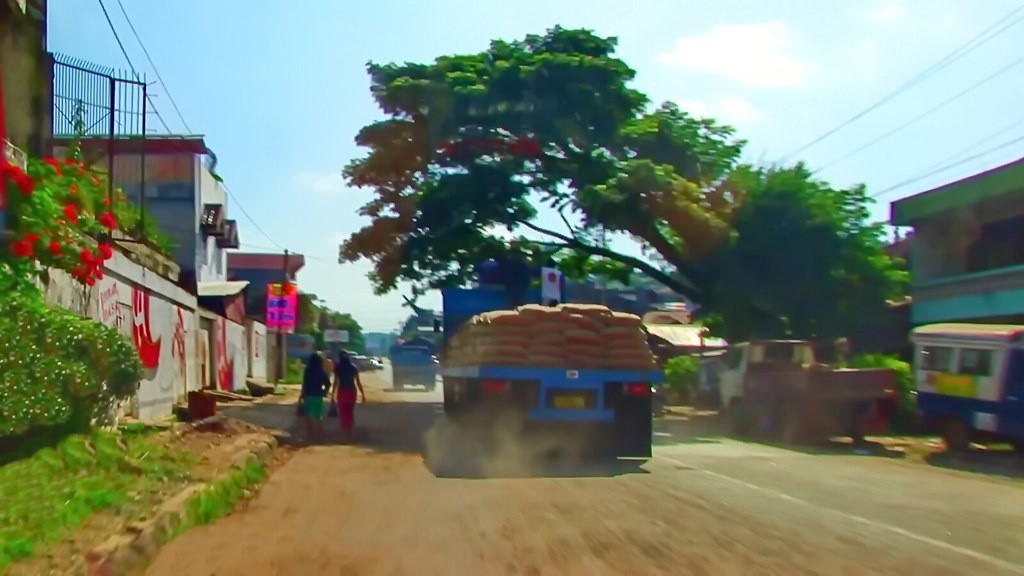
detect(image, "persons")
[297,353,331,440]
[332,350,367,444]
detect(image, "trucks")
[718,339,903,447]
[438,259,666,467]
[909,321,1024,462]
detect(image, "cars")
[348,351,384,372]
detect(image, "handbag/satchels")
[296,399,305,416]
[328,400,337,416]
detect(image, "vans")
[391,345,436,392]
